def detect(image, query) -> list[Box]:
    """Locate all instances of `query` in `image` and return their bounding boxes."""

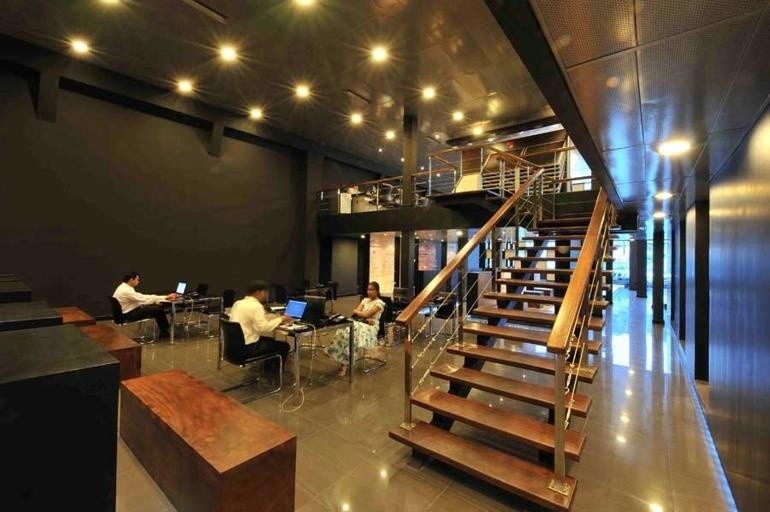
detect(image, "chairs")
[219,301,386,406]
[108,279,237,347]
[362,291,459,345]
[340,183,427,211]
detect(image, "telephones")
[187,291,200,298]
[328,314,346,323]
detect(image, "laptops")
[303,296,332,320]
[280,299,307,324]
[188,282,208,299]
[166,282,187,299]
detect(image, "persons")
[227,282,293,392]
[111,271,179,340]
[320,281,387,380]
